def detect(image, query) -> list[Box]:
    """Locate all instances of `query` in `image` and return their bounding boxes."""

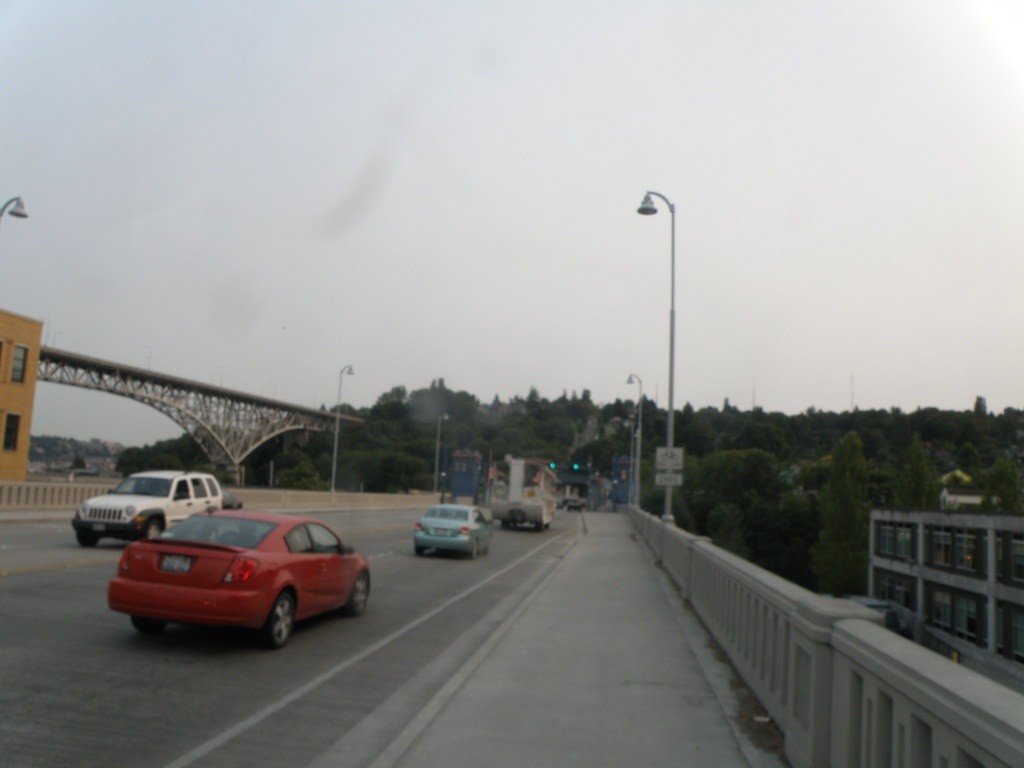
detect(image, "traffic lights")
[572,464,579,470]
[548,463,557,469]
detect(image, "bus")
[488,459,559,531]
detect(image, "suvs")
[72,470,224,548]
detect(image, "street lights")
[331,365,355,490]
[433,413,449,494]
[637,191,675,519]
[627,375,642,505]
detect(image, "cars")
[222,490,243,510]
[413,504,493,560]
[107,509,371,651]
